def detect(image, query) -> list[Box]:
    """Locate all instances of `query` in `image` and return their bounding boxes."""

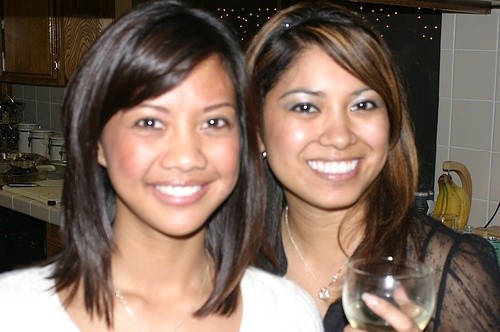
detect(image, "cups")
[16,123,39,153]
[342,257,436,332]
[51,137,67,161]
[31,129,54,161]
[463,226,474,233]
[431,213,458,232]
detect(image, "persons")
[1,0,424,332]
[246,0,500,332]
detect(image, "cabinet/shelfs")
[0,0,135,87]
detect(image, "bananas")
[429,173,471,231]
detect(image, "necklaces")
[285,205,350,300]
[114,291,148,332]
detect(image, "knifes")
[8,183,63,187]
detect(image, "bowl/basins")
[3,153,40,169]
